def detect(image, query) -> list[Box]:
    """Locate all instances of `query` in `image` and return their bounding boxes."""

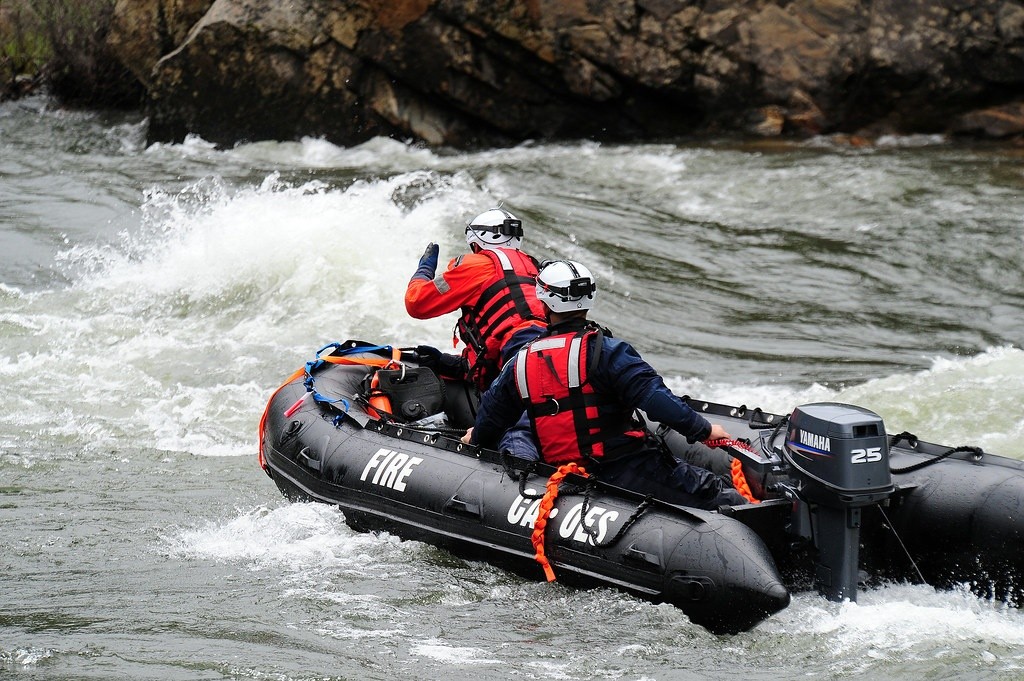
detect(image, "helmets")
[464,202,523,254]
[535,261,597,313]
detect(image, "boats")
[258,341,1024,638]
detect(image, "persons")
[457,264,747,508]
[406,207,550,460]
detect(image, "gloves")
[411,242,439,281]
